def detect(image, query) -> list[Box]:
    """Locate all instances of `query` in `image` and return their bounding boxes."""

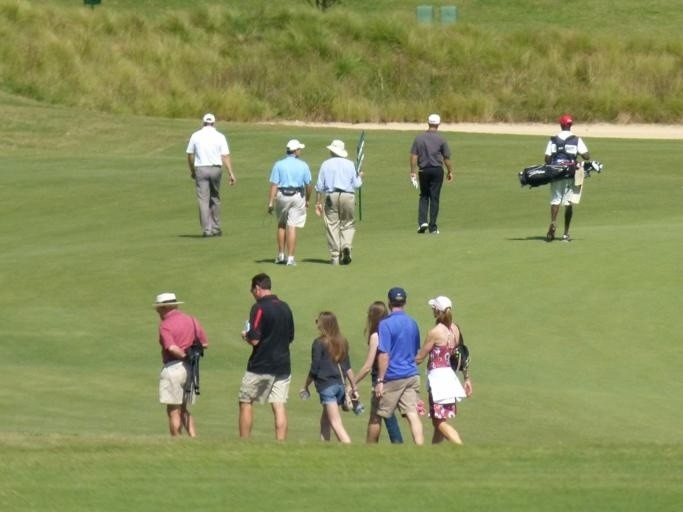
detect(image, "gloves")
[410,175,419,189]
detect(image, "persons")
[544,114,590,240]
[238,272,294,442]
[305,312,359,443]
[315,139,364,264]
[416,296,472,444]
[152,293,207,437]
[346,301,403,443]
[186,113,236,237]
[410,114,454,234]
[268,139,312,266]
[367,287,424,445]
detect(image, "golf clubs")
[584,161,603,173]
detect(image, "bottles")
[352,401,364,416]
[454,348,459,360]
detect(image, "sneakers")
[274,253,298,267]
[330,244,354,266]
[416,222,440,236]
[561,233,570,243]
[546,221,559,241]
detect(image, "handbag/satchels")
[343,383,354,412]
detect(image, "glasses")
[248,288,259,293]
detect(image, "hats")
[203,113,216,124]
[427,113,441,125]
[285,139,305,153]
[150,292,184,308]
[429,296,453,312]
[388,288,407,305]
[326,139,348,157]
[560,114,574,127]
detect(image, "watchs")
[375,378,385,384]
[464,375,470,379]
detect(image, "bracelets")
[352,386,359,392]
